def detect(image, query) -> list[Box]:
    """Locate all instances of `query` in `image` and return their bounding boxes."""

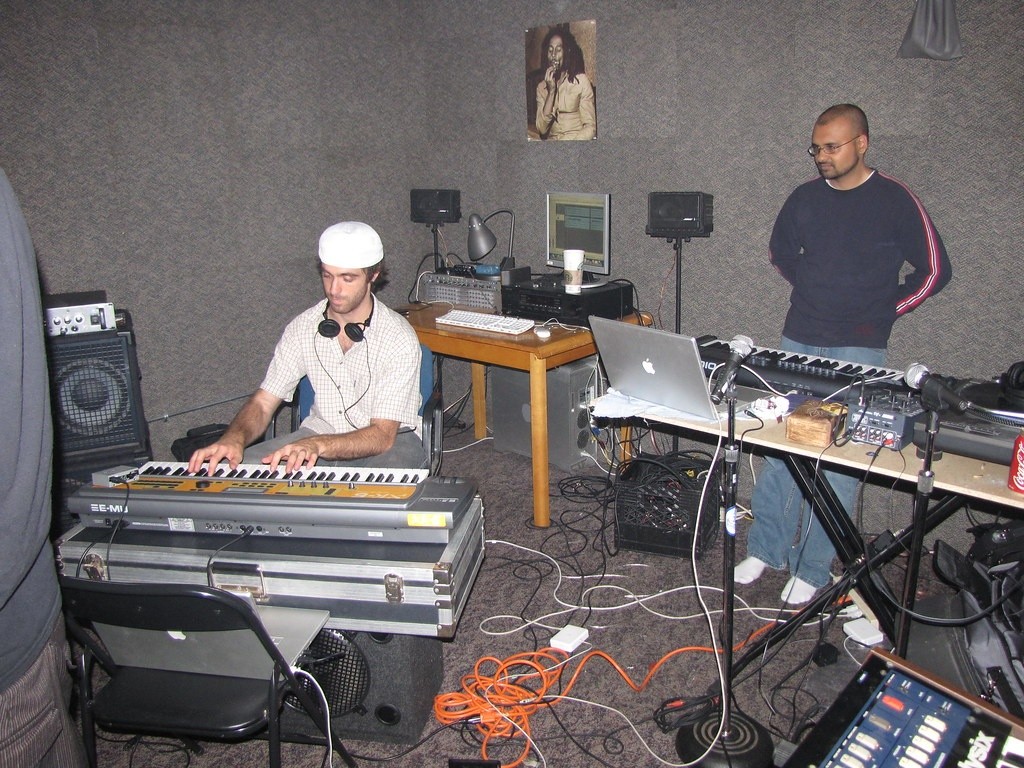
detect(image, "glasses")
[808,136,861,155]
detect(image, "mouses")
[536,327,551,338]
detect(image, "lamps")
[468,208,515,282]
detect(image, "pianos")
[64,458,482,544]
[668,334,905,403]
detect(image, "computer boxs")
[491,357,597,475]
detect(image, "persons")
[536,26,596,140]
[735,106,952,605]
[188,220,422,477]
[0,167,93,768]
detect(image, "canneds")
[1007,428,1024,494]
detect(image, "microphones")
[903,363,969,414]
[710,335,754,405]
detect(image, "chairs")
[59,575,359,768]
[263,346,441,477]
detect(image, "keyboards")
[435,310,535,335]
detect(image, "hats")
[318,221,383,269]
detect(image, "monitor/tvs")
[545,192,612,288]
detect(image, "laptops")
[91,591,331,681]
[588,315,773,421]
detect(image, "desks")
[591,392,1024,701]
[405,305,661,528]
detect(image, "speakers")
[45,308,148,466]
[410,188,462,227]
[645,191,714,243]
[278,628,444,745]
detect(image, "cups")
[562,248,585,294]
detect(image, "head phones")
[318,291,374,342]
[1000,361,1024,406]
